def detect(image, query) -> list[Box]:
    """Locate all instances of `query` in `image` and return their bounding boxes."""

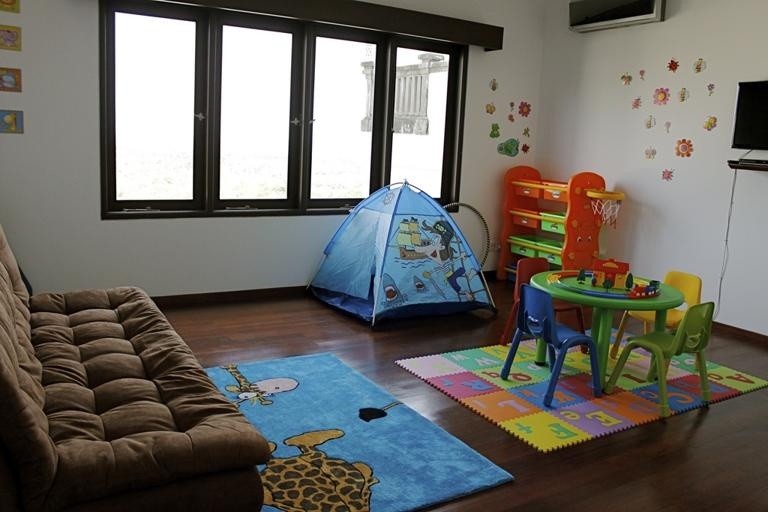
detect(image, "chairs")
[505,254,716,420]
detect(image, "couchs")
[0,227,275,512]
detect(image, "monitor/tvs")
[731,79,768,150]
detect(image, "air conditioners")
[567,0,670,36]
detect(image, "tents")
[305,181,498,327]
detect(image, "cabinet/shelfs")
[494,163,605,287]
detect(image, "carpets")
[199,353,516,512]
